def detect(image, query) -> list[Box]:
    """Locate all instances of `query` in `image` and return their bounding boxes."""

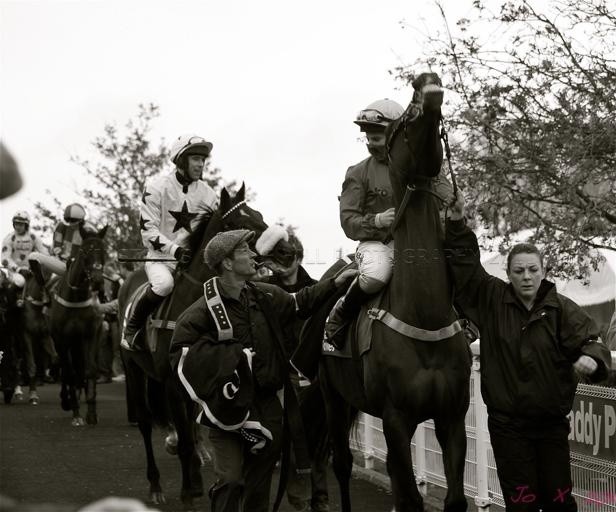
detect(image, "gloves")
[174,247,191,263]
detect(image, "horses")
[10,273,59,406]
[48,222,109,428]
[117,180,297,508]
[308,72,475,512]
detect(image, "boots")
[124,284,166,352]
[326,275,370,349]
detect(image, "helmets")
[170,133,213,164]
[12,210,30,231]
[353,97,404,128]
[64,205,85,222]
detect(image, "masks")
[366,143,386,162]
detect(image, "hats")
[203,229,256,269]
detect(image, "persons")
[120,260,136,278]
[29,203,98,306]
[444,187,612,512]
[124,133,219,352]
[323,100,405,352]
[274,234,319,296]
[0,210,49,279]
[90,288,119,315]
[170,230,360,512]
